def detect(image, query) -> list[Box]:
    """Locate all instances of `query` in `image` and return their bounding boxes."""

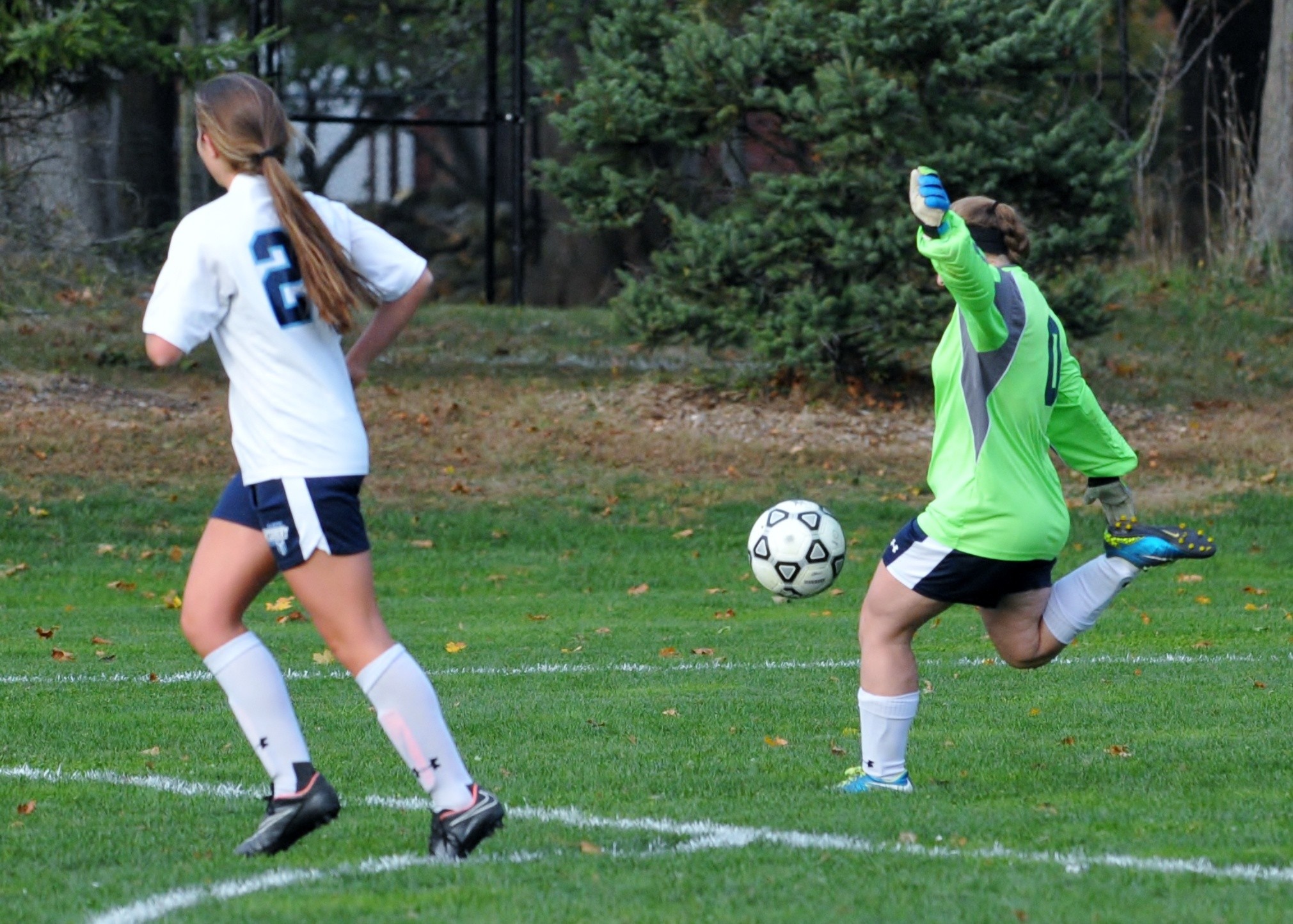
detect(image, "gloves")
[1082,478,1137,527]
[907,167,952,239]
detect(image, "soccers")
[747,497,848,601]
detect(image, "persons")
[820,165,1220,789]
[140,72,506,863]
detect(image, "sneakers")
[1101,516,1219,574]
[821,765,916,797]
[229,770,343,860]
[426,783,506,860]
